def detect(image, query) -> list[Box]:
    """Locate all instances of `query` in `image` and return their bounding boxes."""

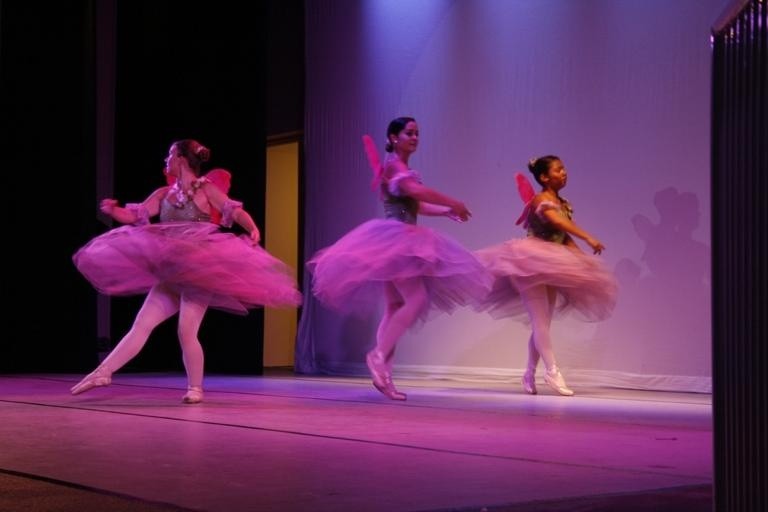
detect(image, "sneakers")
[543,372,574,395]
[70,371,112,395]
[521,373,537,394]
[366,350,407,401]
[182,388,203,403]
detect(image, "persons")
[505,151,607,396]
[68,138,264,404]
[362,116,473,401]
